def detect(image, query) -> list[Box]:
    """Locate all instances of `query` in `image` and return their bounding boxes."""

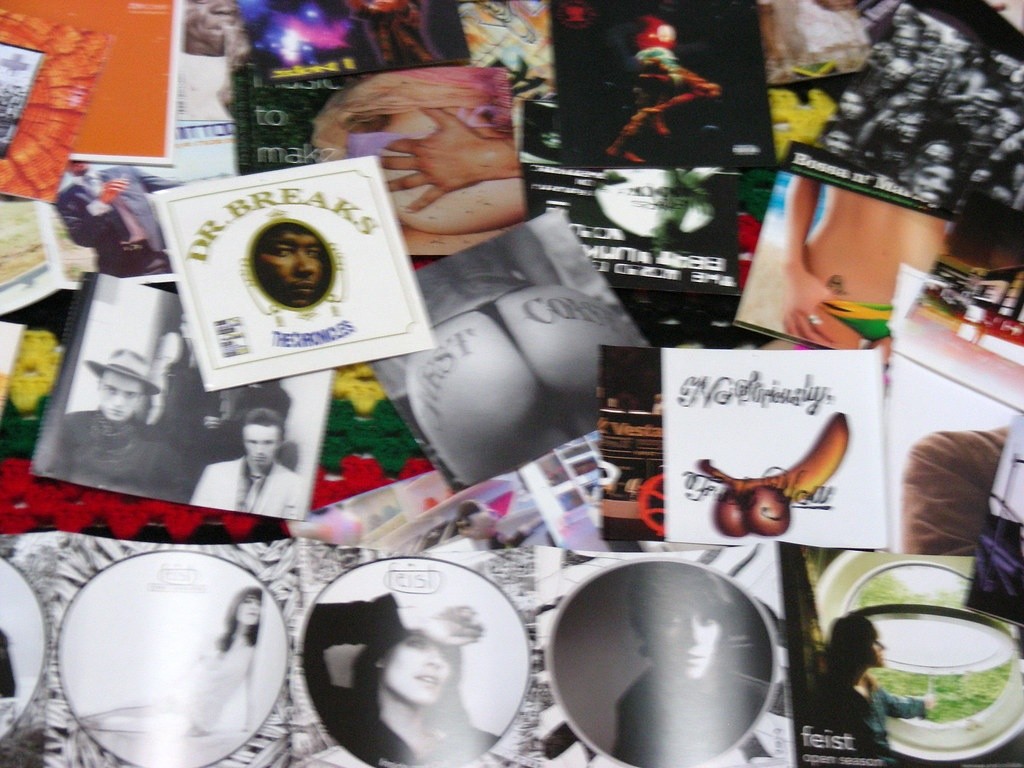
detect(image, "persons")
[0,0,1024,768]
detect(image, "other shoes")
[648,119,671,136]
[607,145,647,165]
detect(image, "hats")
[84,349,161,396]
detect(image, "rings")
[807,314,822,325]
